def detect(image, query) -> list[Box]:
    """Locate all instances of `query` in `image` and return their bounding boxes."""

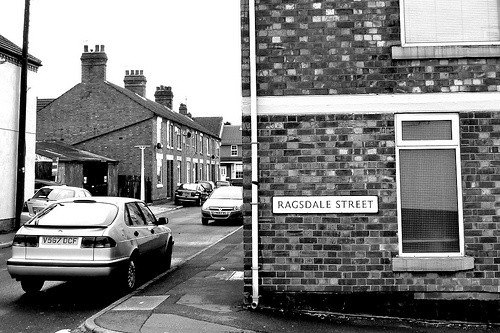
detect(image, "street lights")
[134,145,150,201]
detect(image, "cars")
[19,186,91,227]
[198,180,216,190]
[201,186,243,225]
[215,180,232,189]
[175,183,208,207]
[7,196,174,294]
[195,181,213,197]
